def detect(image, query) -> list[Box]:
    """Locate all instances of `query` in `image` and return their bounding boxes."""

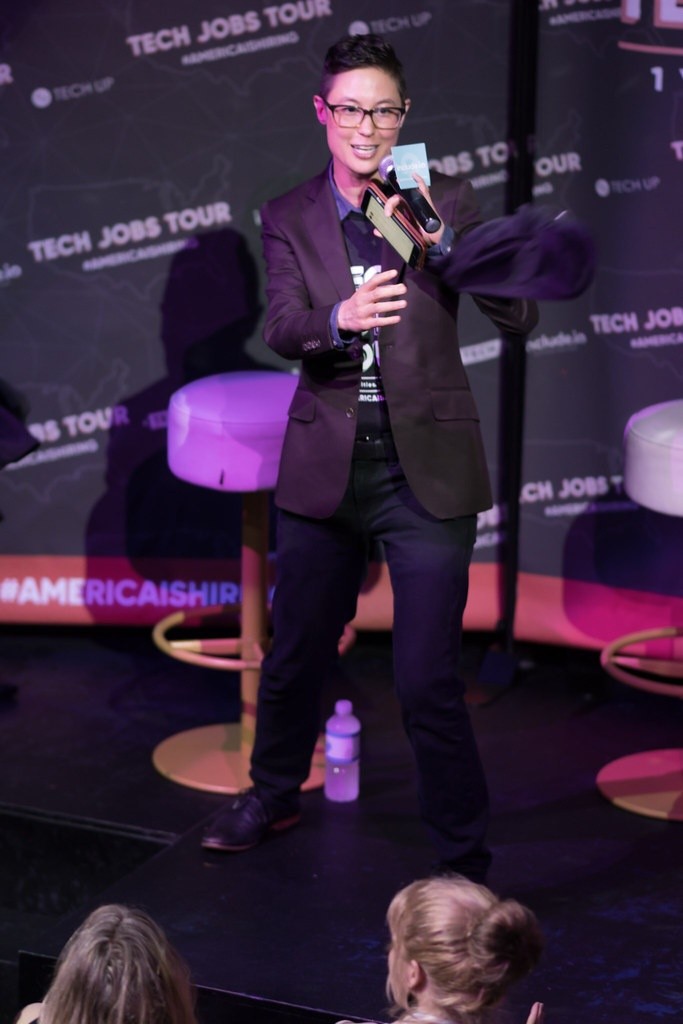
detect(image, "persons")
[16,904,194,1024]
[199,33,536,851]
[335,874,544,1024]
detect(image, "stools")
[595,397,683,824]
[153,369,328,793]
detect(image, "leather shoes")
[199,787,302,850]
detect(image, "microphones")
[379,155,442,233]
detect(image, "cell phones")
[358,178,428,273]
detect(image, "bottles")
[324,700,361,800]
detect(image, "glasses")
[319,94,406,130]
